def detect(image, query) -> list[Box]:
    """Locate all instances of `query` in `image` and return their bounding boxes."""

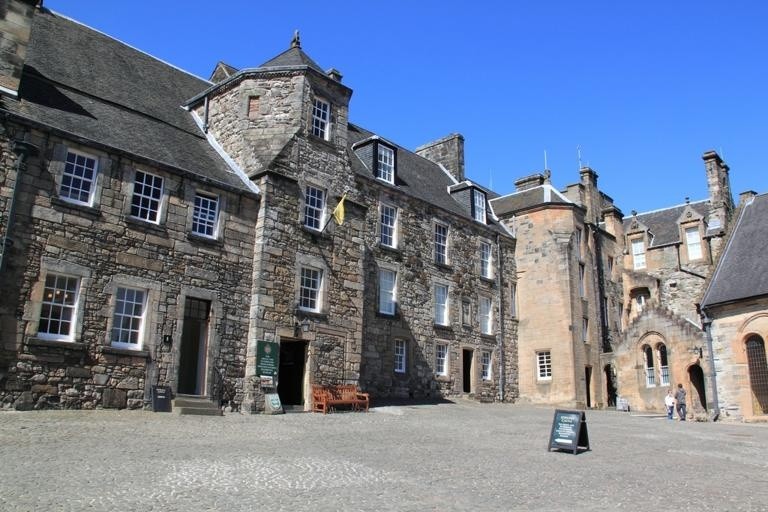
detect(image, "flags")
[333,194,347,226]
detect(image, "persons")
[665,390,675,419]
[675,383,686,421]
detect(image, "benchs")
[310,384,370,414]
